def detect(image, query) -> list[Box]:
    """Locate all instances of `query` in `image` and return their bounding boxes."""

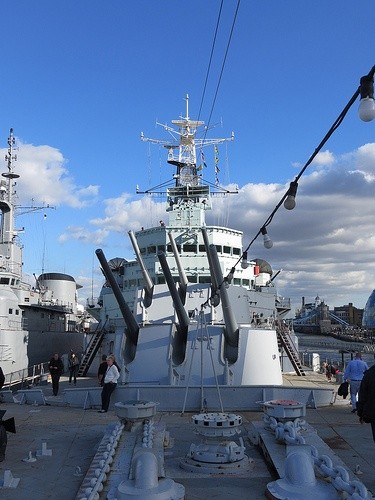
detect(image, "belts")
[351,379,362,381]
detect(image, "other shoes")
[98,410,107,413]
[351,409,357,413]
[74,382,76,385]
[69,381,71,384]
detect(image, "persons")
[68,353,79,384]
[343,351,369,413]
[321,362,337,377]
[356,353,375,443]
[97,355,120,413]
[48,353,64,396]
[98,353,121,387]
